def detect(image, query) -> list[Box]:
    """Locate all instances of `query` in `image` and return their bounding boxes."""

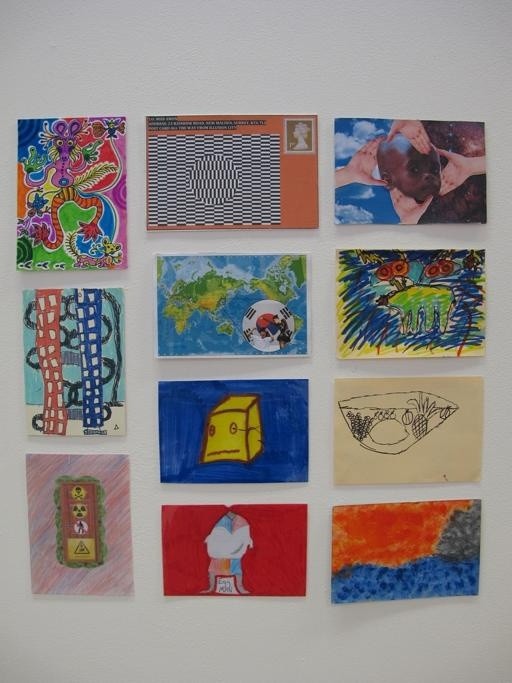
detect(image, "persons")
[335,135,389,187]
[388,187,433,223]
[386,119,430,156]
[438,147,486,195]
